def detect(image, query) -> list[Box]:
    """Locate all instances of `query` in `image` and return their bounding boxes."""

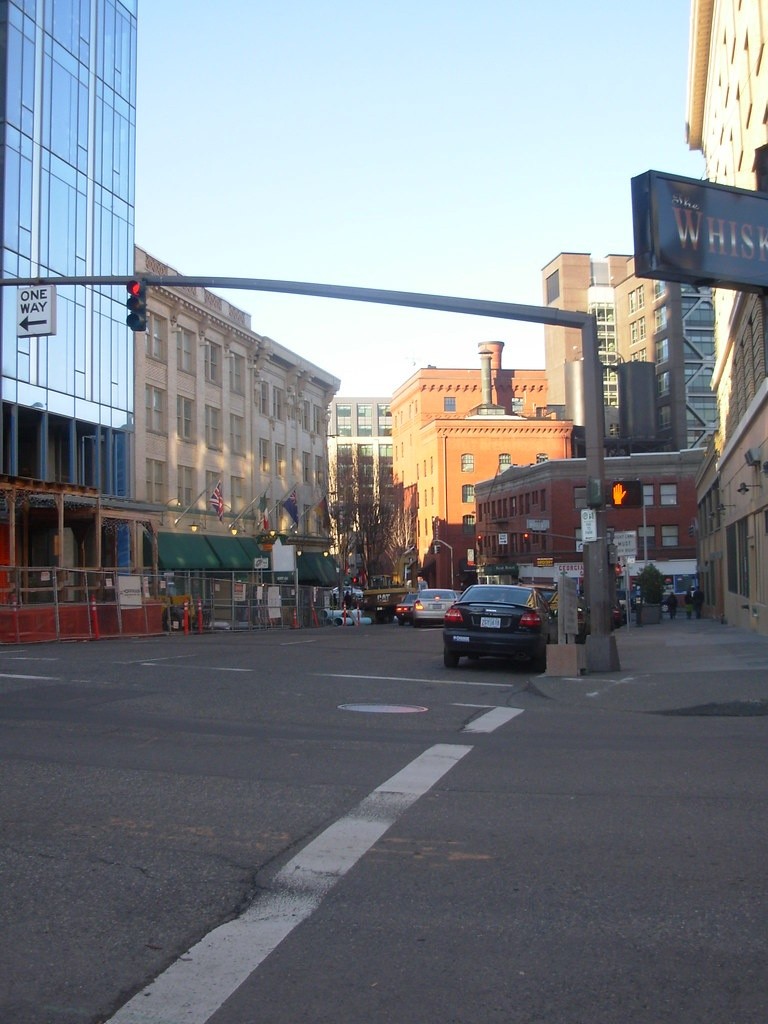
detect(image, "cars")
[330,586,363,598]
[610,598,624,628]
[517,583,590,643]
[616,589,631,617]
[396,589,463,628]
[444,585,558,673]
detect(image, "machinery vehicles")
[363,574,416,624]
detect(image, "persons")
[684,591,694,619]
[666,592,678,620]
[329,594,337,606]
[344,591,351,607]
[693,585,704,619]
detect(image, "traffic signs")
[16,284,56,338]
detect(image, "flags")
[283,485,299,527]
[257,484,270,533]
[207,471,226,526]
[314,494,333,535]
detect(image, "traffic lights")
[127,278,147,331]
[478,535,482,544]
[523,533,529,543]
[611,480,644,508]
[431,545,440,554]
